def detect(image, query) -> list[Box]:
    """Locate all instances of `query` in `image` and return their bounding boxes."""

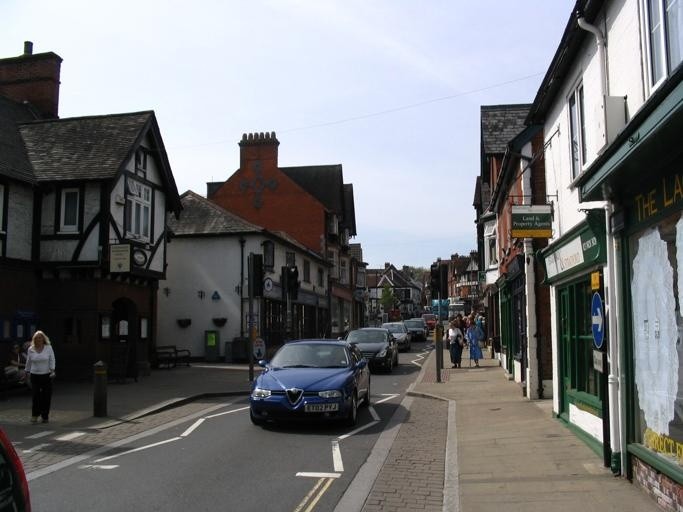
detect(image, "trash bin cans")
[225,337,248,363]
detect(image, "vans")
[422,314,438,330]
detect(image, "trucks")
[431,299,450,321]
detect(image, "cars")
[336,327,399,373]
[248,338,371,426]
[403,317,429,342]
[380,321,412,353]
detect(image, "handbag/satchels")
[454,334,467,347]
[479,341,484,348]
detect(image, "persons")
[445,307,486,369]
[0,337,33,387]
[24,330,56,425]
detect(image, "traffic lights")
[427,263,449,300]
[287,265,301,299]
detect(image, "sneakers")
[452,365,461,368]
[30,417,49,424]
